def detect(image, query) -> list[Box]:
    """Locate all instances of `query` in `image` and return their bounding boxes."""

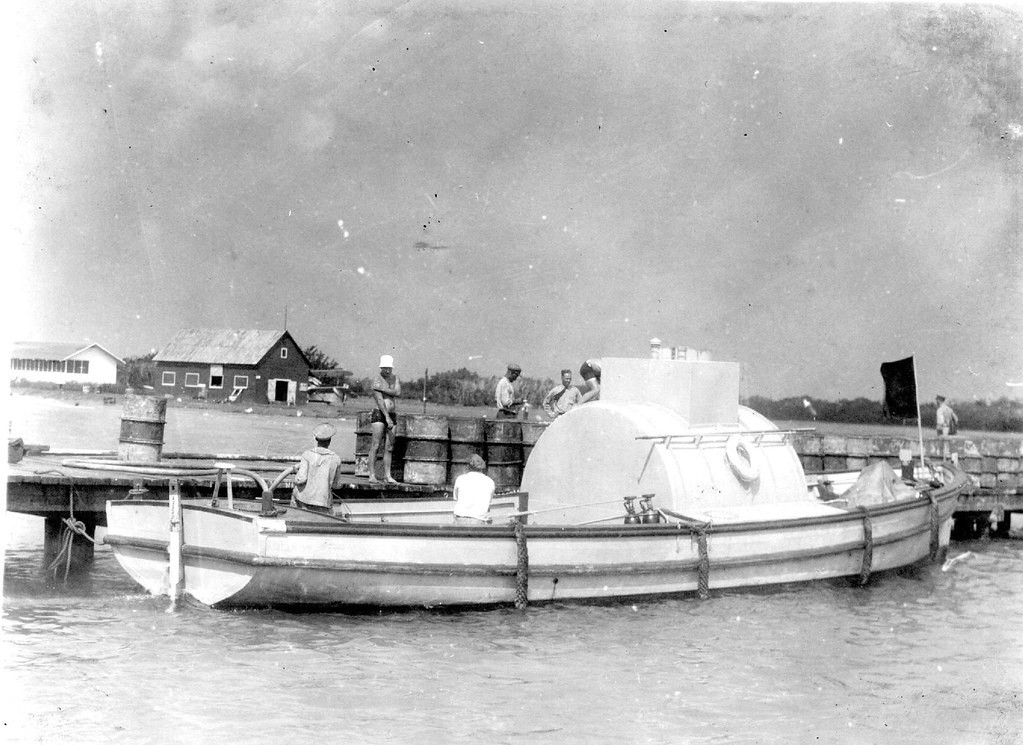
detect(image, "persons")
[495,363,523,419]
[543,369,582,421]
[369,355,401,483]
[294,422,341,516]
[453,453,495,526]
[935,395,958,435]
[802,395,818,421]
[575,359,601,405]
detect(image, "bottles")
[522,399,529,421]
[650,337,662,359]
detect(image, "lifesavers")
[725,434,762,484]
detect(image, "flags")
[880,356,919,419]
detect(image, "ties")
[553,387,567,400]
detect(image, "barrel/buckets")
[485,420,524,493]
[354,412,394,474]
[390,413,407,480]
[790,430,824,475]
[448,417,487,484]
[405,414,448,484]
[117,394,169,463]
[522,422,552,467]
[822,434,1022,489]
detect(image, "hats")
[379,355,393,368]
[508,363,522,378]
[935,395,946,402]
[313,422,337,440]
[467,453,486,469]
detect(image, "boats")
[100,348,970,612]
